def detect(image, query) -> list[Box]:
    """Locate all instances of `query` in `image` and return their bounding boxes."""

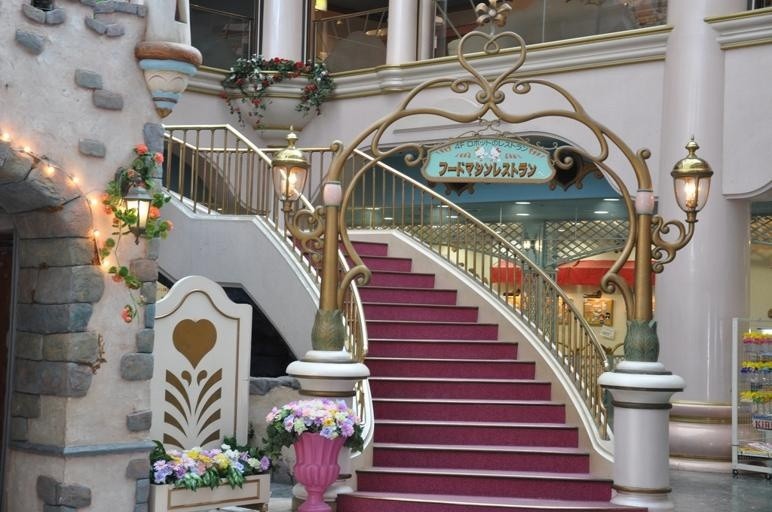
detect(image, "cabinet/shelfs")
[730,315,772,479]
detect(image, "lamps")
[314,0,329,12]
[272,125,324,255]
[120,173,156,244]
[650,134,713,274]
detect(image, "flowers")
[98,145,176,325]
[152,434,271,491]
[267,396,364,459]
[220,54,337,127]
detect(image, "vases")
[294,432,346,512]
[149,473,271,512]
[227,71,321,129]
